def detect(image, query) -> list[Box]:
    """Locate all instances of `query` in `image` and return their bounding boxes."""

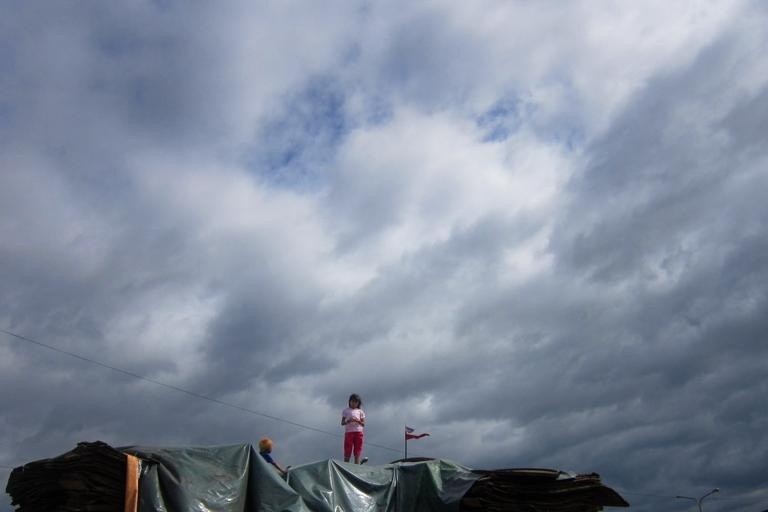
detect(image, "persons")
[341,394,365,465]
[259,438,284,472]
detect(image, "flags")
[406,427,414,433]
[406,434,429,439]
